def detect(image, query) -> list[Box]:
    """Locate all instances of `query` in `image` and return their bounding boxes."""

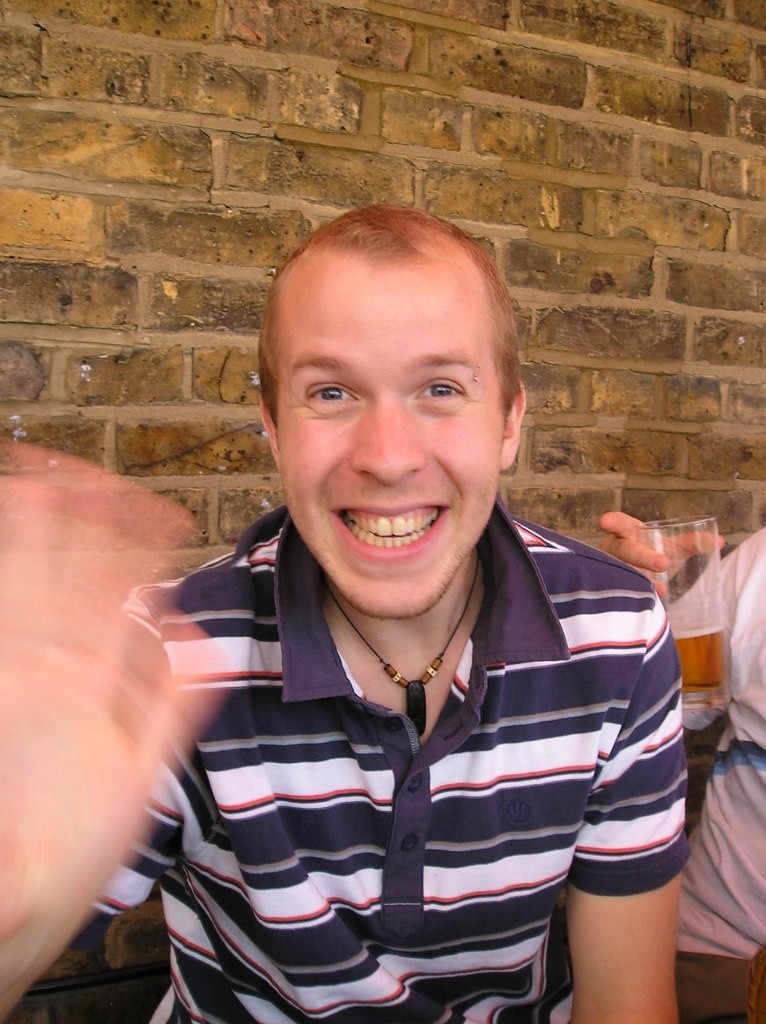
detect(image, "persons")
[0,203,691,1024]
[594,497,766,1024]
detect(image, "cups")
[637,515,733,710]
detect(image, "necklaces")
[321,549,480,738]
